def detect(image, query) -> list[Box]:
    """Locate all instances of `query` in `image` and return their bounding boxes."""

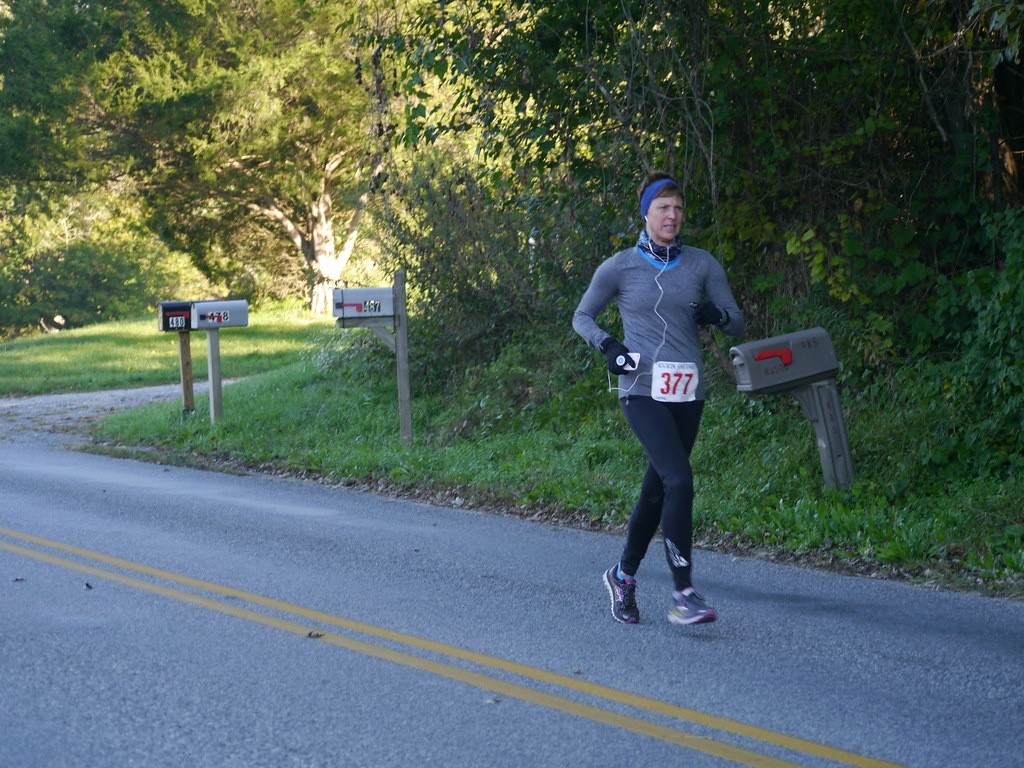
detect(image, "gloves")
[599,337,636,375]
[689,301,722,329]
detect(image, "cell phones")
[624,353,640,370]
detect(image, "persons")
[570,173,744,624]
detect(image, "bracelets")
[715,306,727,325]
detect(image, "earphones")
[644,216,649,222]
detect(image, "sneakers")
[667,590,717,625]
[602,564,640,624]
